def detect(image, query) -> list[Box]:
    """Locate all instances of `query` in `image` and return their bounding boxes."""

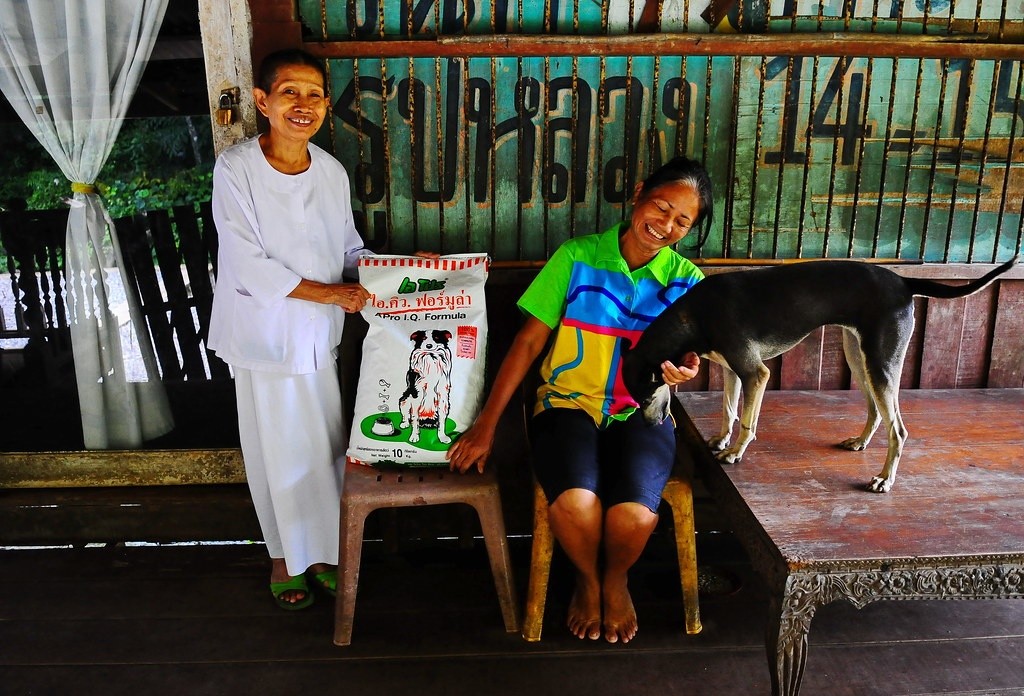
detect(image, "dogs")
[621,252,1022,494]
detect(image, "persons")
[446,157,716,643]
[206,48,439,610]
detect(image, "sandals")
[315,567,338,598]
[269,573,315,610]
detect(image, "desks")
[670,387,1024,696]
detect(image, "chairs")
[332,460,520,648]
[520,376,703,643]
